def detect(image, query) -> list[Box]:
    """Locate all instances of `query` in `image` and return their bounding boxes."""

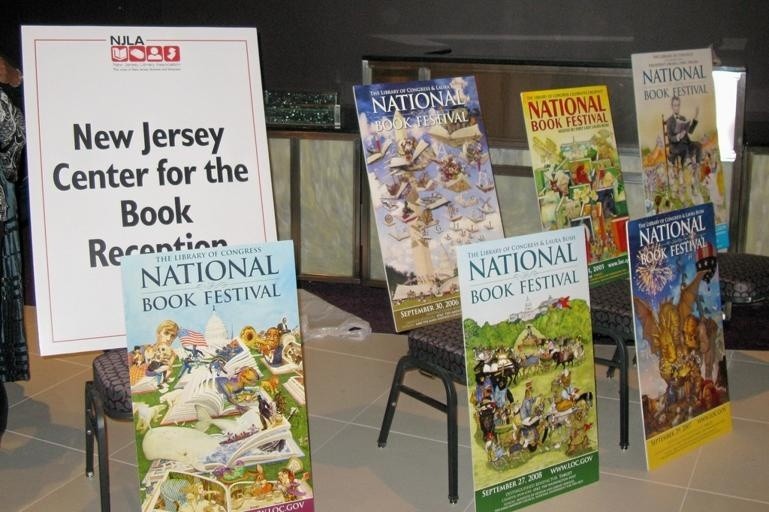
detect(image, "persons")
[0,56,24,97]
[132,320,206,385]
[256,392,273,429]
[261,318,291,367]
[177,480,222,512]
[668,97,702,168]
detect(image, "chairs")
[719,252,768,308]
[589,278,635,451]
[377,316,468,503]
[85,349,134,512]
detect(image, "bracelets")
[10,66,22,87]
